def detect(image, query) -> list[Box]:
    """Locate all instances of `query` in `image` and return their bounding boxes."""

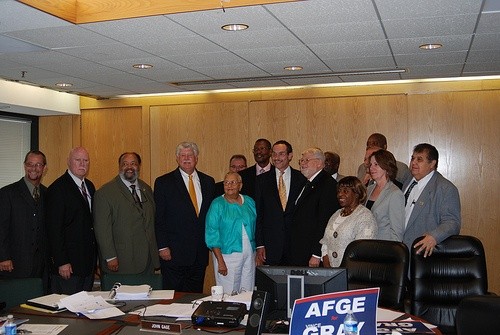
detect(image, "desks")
[0,292,442,335]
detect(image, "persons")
[0,149,50,313]
[323,152,346,183]
[46,146,98,295]
[238,138,273,197]
[213,154,249,197]
[401,143,461,281]
[91,151,160,291]
[255,140,308,265]
[289,147,343,267]
[204,172,258,295]
[319,176,377,268]
[153,141,215,294]
[358,133,410,243]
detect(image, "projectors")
[191,301,247,327]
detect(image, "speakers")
[245,289,270,335]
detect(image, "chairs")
[407,235,497,335]
[338,239,410,313]
[455,295,500,335]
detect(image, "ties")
[81,182,88,203]
[404,181,417,206]
[260,169,265,174]
[297,180,311,204]
[278,171,287,211]
[33,186,38,201]
[188,174,198,216]
[130,185,141,209]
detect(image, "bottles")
[4,315,17,335]
[344,310,358,335]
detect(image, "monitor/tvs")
[256,269,348,334]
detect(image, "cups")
[211,285,223,301]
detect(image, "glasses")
[299,159,321,165]
[223,180,241,186]
[25,163,44,168]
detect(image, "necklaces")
[340,207,356,217]
[225,194,239,200]
